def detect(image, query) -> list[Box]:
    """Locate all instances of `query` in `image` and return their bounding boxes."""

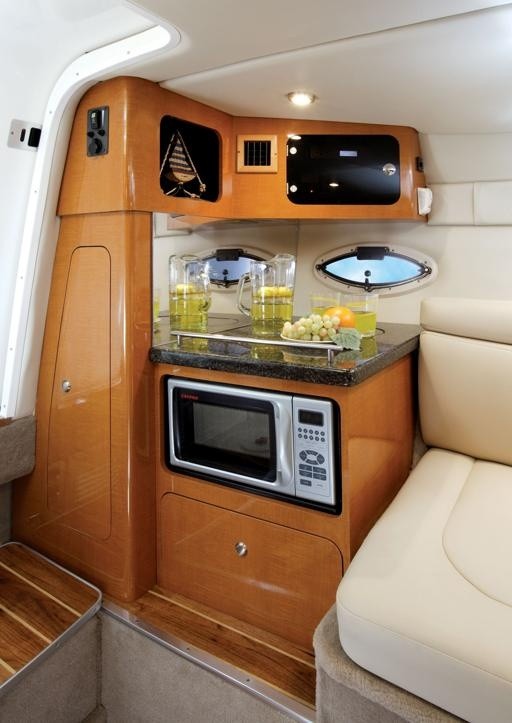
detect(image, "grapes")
[283,354,329,367]
[282,313,340,342]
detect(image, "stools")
[2,538,109,723]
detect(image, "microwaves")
[164,378,338,513]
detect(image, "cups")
[168,252,211,333]
[308,291,340,314]
[342,293,378,337]
[237,253,297,337]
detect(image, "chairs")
[309,293,511,721]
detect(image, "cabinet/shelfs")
[11,208,160,603]
[156,490,405,651]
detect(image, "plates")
[281,333,335,345]
